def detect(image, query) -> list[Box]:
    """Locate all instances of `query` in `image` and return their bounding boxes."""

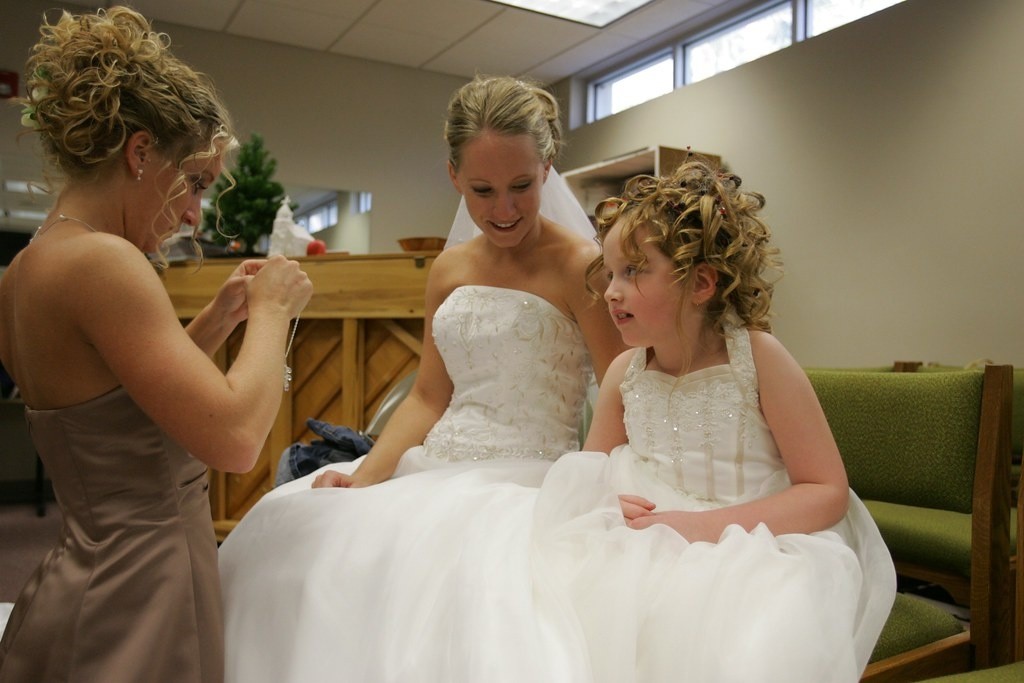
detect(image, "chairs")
[800,364,1014,683]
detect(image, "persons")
[217,72,632,683]
[530,158,900,683]
[0,4,316,683]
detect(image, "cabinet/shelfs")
[559,145,721,228]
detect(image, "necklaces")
[282,312,301,392]
[30,215,98,246]
